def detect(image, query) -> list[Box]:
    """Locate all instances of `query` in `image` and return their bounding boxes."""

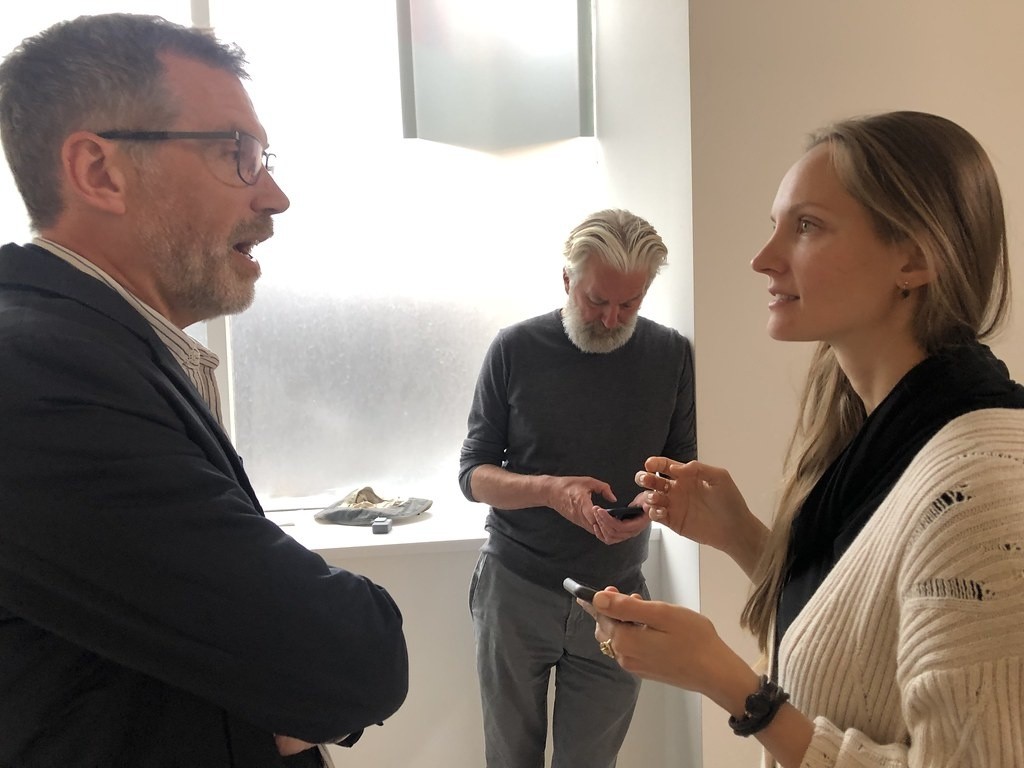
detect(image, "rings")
[599,621,617,659]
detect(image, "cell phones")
[603,507,645,516]
[563,577,604,601]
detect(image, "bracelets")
[728,674,790,738]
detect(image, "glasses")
[95,127,276,186]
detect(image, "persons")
[0,12,412,767]
[455,207,699,768]
[570,108,1020,767]
